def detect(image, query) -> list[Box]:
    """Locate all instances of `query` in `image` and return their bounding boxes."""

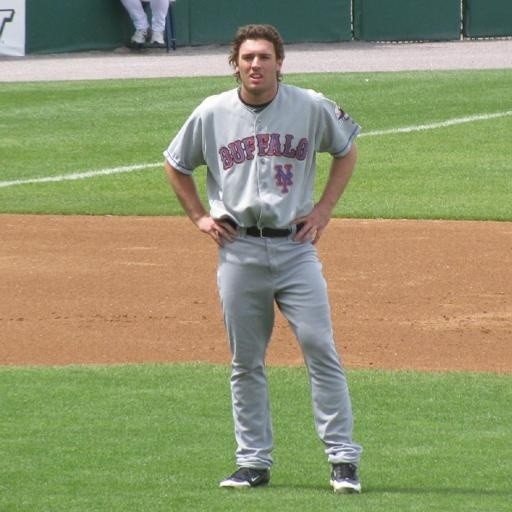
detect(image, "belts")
[246,225,303,238]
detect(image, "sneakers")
[329,463,362,492]
[219,469,270,489]
[149,31,165,44]
[130,29,148,44]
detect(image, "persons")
[162,20,371,495]
[120,0,172,47]
